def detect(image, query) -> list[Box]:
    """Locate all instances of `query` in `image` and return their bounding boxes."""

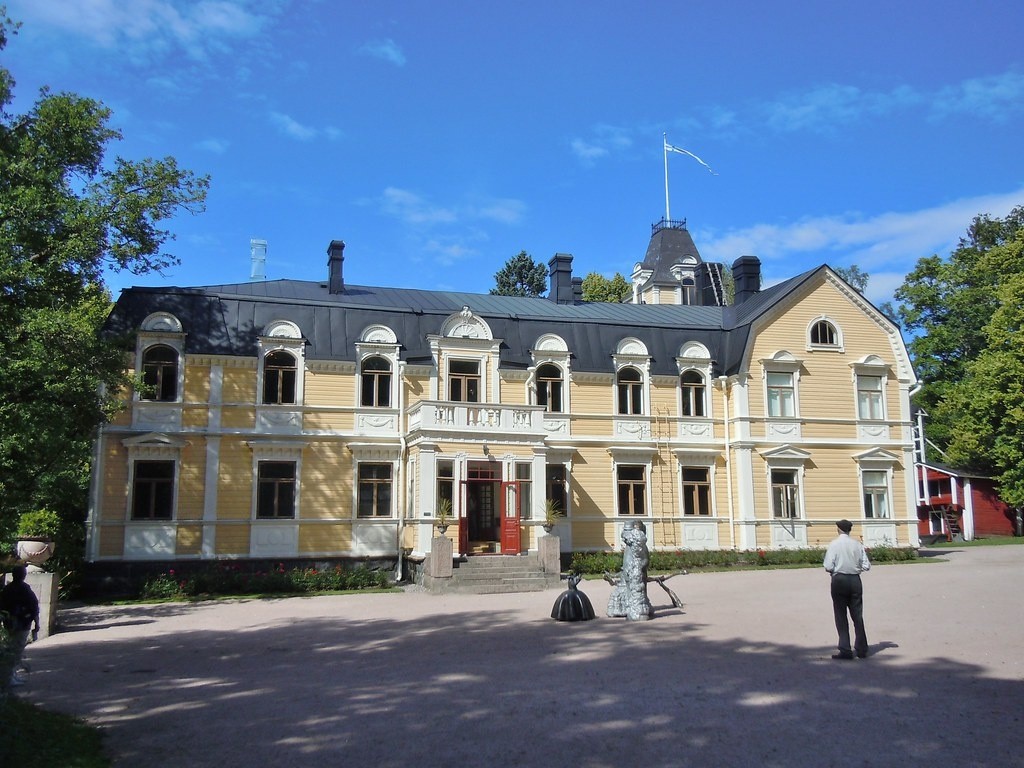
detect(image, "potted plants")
[538,499,565,532]
[16,508,62,565]
[435,496,453,535]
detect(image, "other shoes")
[9,677,25,686]
[858,651,867,658]
[831,654,852,659]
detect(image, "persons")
[823,519,871,661]
[1,566,40,669]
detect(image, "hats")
[836,519,853,533]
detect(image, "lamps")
[484,444,490,455]
